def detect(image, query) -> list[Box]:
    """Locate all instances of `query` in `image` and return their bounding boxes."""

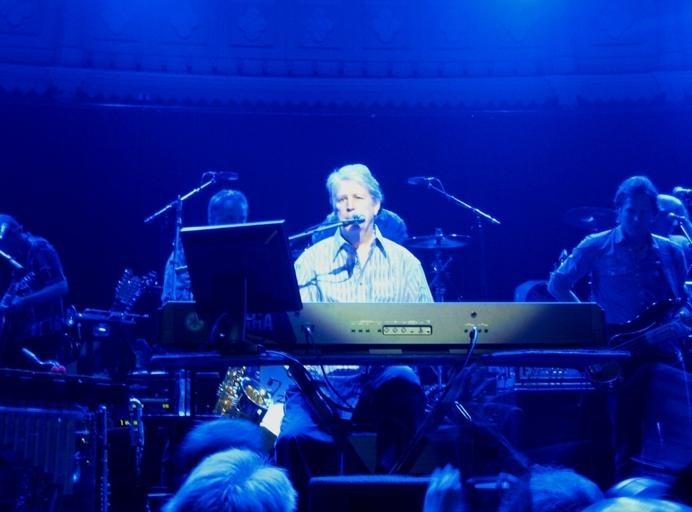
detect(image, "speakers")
[496,387,603,478]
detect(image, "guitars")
[586,282,692,380]
[0,270,35,333]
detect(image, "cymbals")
[562,205,617,227]
[380,183,467,212]
[402,235,474,249]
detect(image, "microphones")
[408,176,426,185]
[353,213,365,223]
[668,213,686,222]
[210,172,237,181]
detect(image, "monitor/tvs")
[180,220,303,342]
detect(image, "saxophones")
[214,366,272,426]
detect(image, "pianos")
[272,301,605,345]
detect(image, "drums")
[259,365,318,437]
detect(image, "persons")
[548,176,692,370]
[274,164,435,512]
[425,464,692,512]
[161,446,298,512]
[160,190,248,303]
[0,216,69,372]
